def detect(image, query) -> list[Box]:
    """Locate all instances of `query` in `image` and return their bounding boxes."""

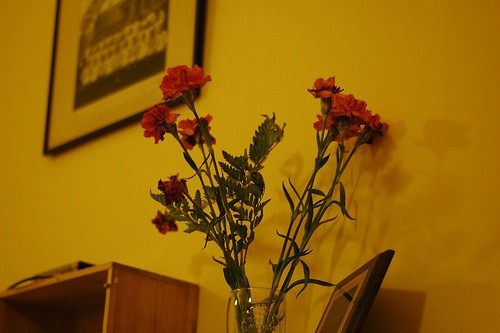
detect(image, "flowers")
[131,67,390,288]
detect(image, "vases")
[224,288,288,333]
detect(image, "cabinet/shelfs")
[0,260,199,333]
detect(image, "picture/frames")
[42,0,207,158]
[314,249,395,332]
